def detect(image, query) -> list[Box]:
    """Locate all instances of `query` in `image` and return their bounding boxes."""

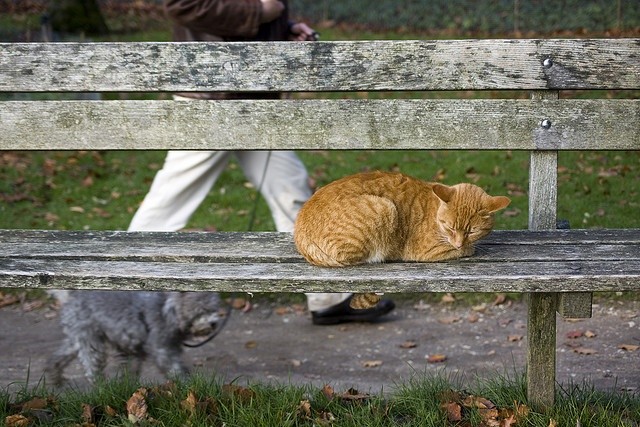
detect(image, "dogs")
[43,286,222,387]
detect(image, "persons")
[126,0,396,325]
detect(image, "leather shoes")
[311,293,395,325]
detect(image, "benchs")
[0,37,640,410]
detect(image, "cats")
[292,167,511,309]
